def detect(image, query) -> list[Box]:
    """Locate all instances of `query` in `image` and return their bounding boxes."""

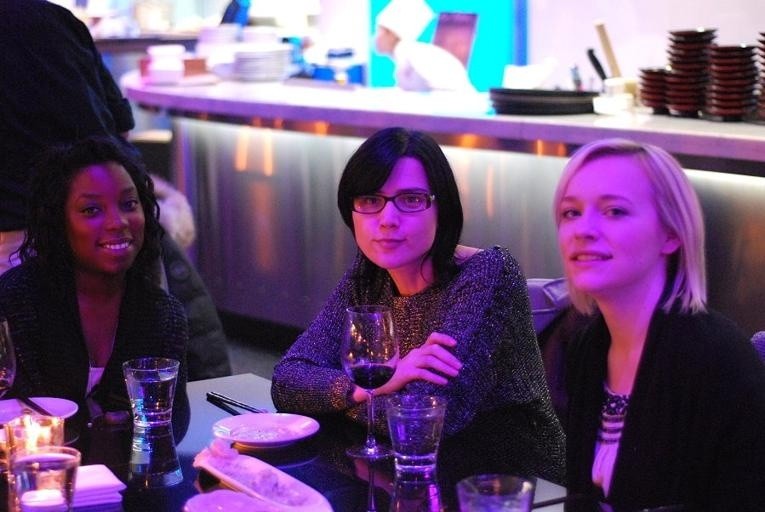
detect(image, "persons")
[0,0,135,226]
[1,134,191,436]
[373,25,481,92]
[535,137,764,512]
[270,127,566,488]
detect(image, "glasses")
[349,193,436,215]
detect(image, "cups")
[123,424,184,491]
[457,474,535,512]
[122,358,180,426]
[385,393,448,475]
[7,415,66,455]
[390,476,443,512]
[8,446,82,512]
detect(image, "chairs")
[521,278,600,414]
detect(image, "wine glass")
[340,303,400,460]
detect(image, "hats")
[376,0,432,43]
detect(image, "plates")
[196,25,293,81]
[490,88,601,116]
[212,412,320,449]
[639,28,765,122]
[0,397,79,425]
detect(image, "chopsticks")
[206,391,262,413]
[0,380,52,416]
